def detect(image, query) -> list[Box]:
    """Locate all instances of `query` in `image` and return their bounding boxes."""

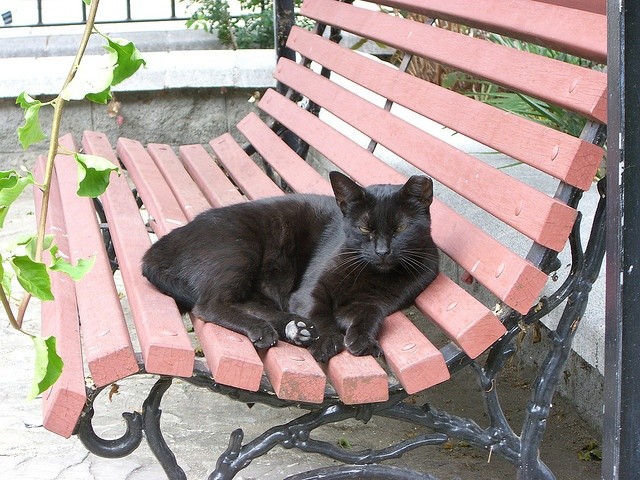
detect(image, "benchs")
[22,0,608,479]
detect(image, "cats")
[141,170,440,363]
